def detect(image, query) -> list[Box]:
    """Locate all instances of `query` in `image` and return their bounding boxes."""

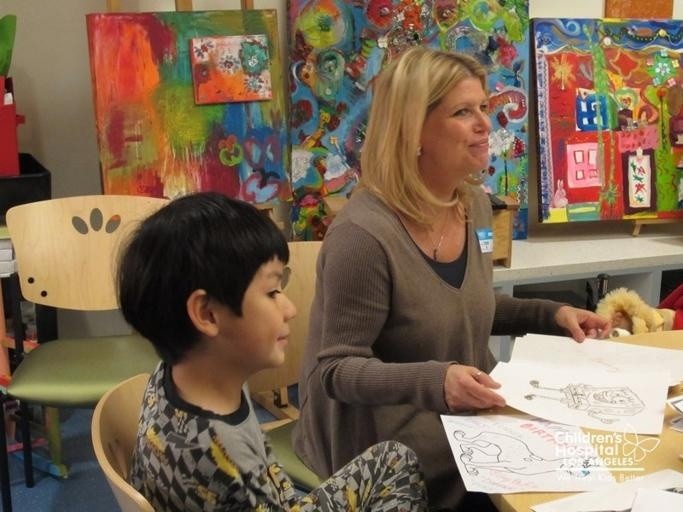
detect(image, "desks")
[492,234,683,309]
[477,330,683,512]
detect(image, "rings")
[471,368,484,381]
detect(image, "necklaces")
[417,202,452,270]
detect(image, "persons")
[292,46,613,512]
[111,188,427,512]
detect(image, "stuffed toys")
[591,284,683,340]
